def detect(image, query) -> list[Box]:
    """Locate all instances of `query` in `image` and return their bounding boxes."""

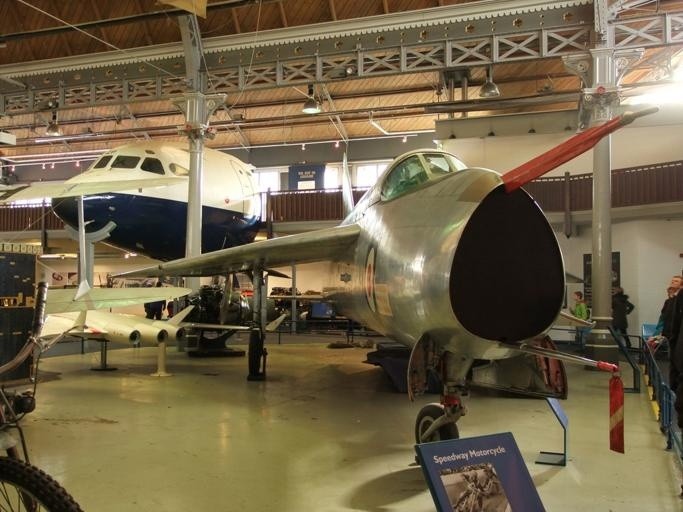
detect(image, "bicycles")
[0,283,87,512]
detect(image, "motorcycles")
[454,468,499,512]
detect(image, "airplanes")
[107,103,661,464]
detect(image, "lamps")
[300,81,323,116]
[476,64,502,100]
[44,106,64,140]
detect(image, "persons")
[656,269,683,429]
[574,291,590,355]
[453,471,484,512]
[143,281,166,320]
[612,288,635,347]
